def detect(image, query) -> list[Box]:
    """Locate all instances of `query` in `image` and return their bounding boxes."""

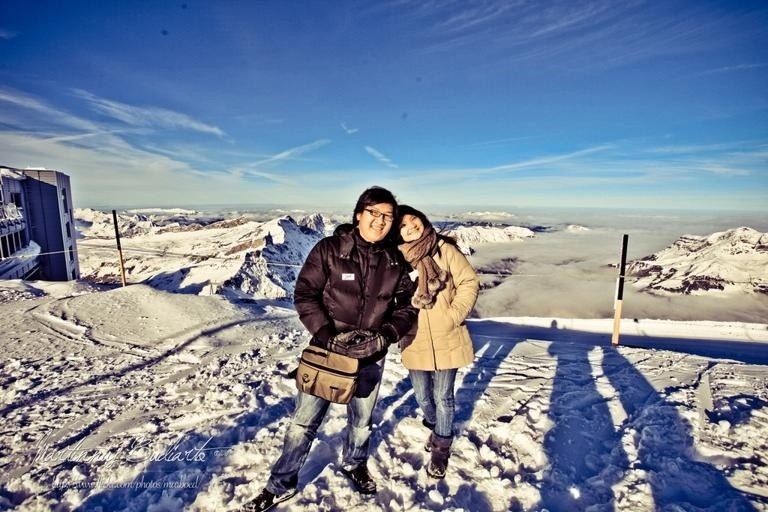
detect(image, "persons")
[390,205,480,479]
[241,186,419,511]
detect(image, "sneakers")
[242,487,297,512]
[341,464,376,495]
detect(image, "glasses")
[364,209,394,221]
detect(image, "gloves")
[326,328,387,358]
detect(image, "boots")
[422,417,453,479]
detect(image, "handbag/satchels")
[295,346,359,404]
